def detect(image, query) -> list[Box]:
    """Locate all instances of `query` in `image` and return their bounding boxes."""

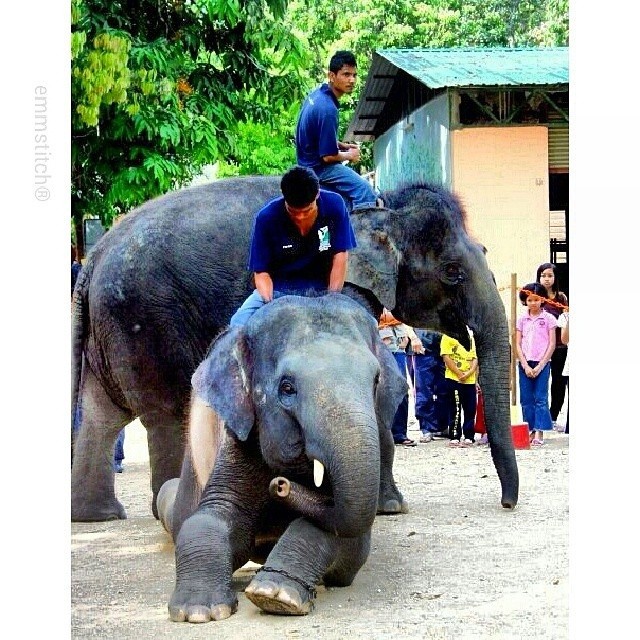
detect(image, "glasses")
[540,274,556,278]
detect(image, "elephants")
[71,173,520,524]
[156,285,410,625]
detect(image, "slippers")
[393,439,417,446]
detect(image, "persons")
[516,282,559,447]
[377,307,419,448]
[404,324,457,440]
[558,291,569,434]
[440,331,477,445]
[113,427,126,474]
[474,383,488,444]
[295,50,379,214]
[228,164,359,350]
[71,244,83,299]
[536,263,568,431]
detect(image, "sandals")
[532,439,544,446]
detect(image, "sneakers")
[449,439,459,446]
[478,434,488,445]
[464,438,472,445]
[420,432,432,443]
